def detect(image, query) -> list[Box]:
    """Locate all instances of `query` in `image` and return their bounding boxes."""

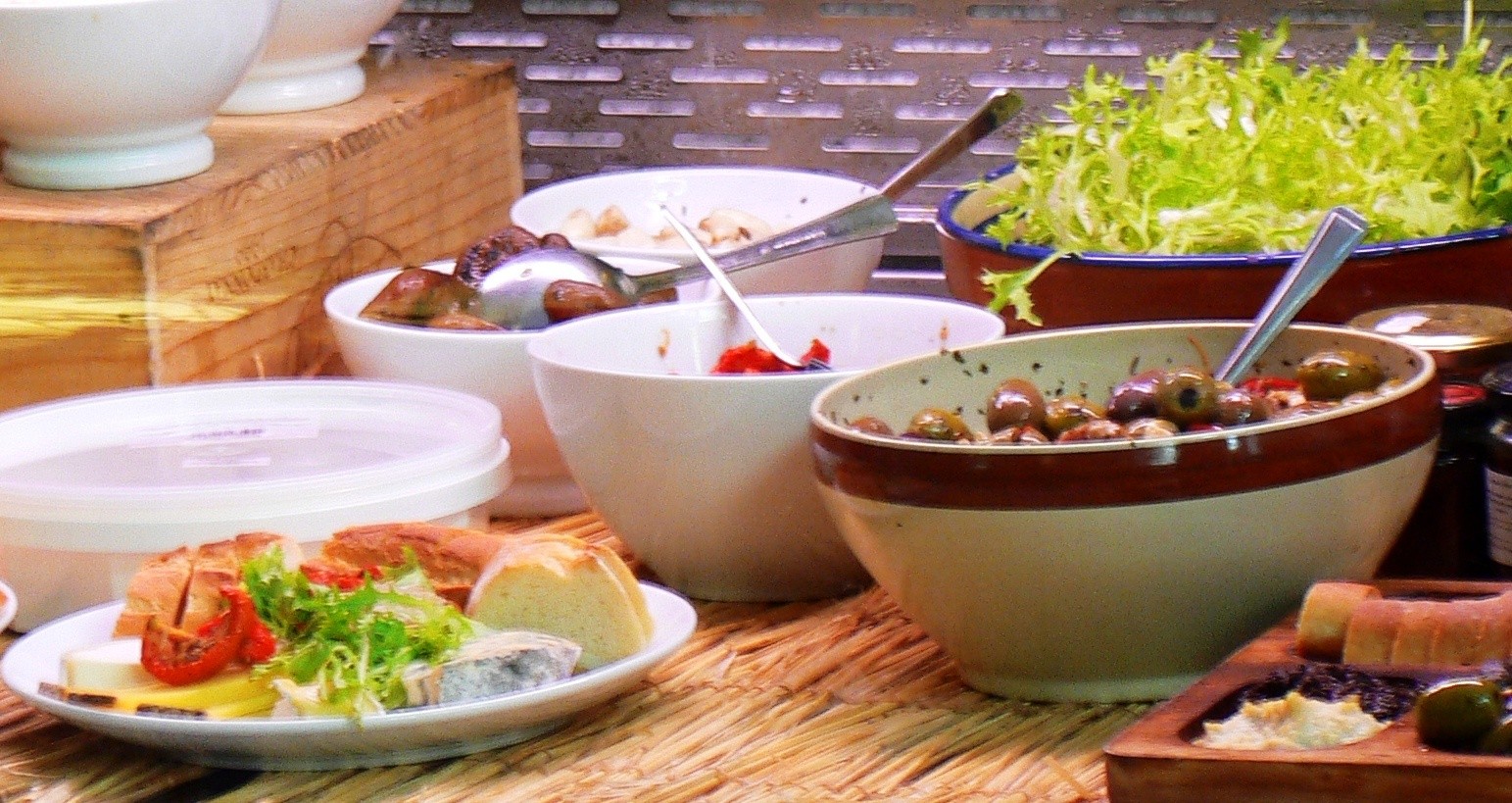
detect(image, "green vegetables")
[955,1,1512,328]
[240,541,474,712]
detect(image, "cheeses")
[37,642,282,719]
[401,628,582,706]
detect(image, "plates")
[1,581,697,770]
[0,582,15,637]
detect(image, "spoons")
[467,197,899,331]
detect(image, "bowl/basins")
[508,165,883,298]
[216,0,400,116]
[809,321,1443,703]
[1,380,513,631]
[934,160,1512,338]
[525,292,1006,601]
[325,257,722,515]
[1,2,284,190]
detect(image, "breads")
[112,523,501,638]
[465,529,654,667]
[1294,581,1511,670]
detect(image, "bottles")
[1482,383,1512,582]
[1404,378,1485,581]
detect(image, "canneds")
[1342,304,1512,578]
